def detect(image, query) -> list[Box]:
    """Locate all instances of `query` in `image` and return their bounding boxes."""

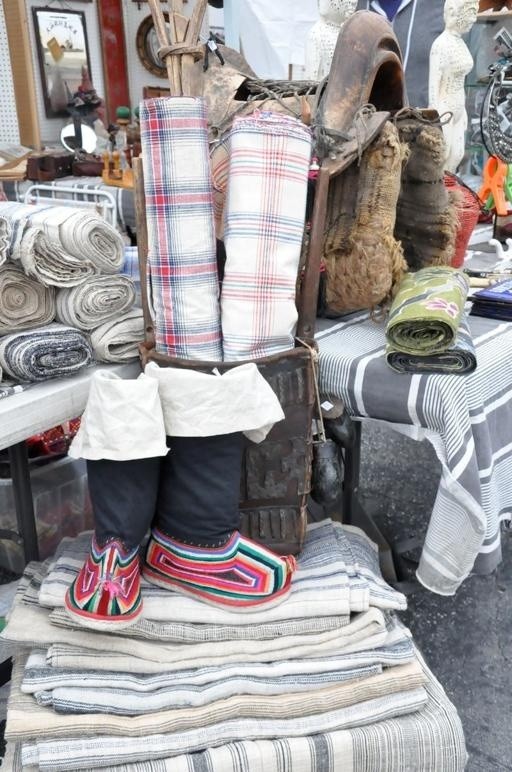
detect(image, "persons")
[304,0,366,83]
[426,0,480,178]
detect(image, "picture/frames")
[30,5,92,121]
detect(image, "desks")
[295,239,512,595]
[0,172,135,247]
[0,356,146,584]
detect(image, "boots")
[63,369,170,633]
[140,360,297,614]
